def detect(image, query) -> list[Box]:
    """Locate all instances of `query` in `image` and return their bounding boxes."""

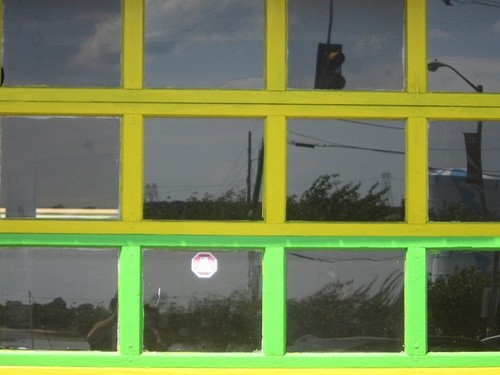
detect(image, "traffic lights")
[314,42,346,94]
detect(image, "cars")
[287,332,487,359]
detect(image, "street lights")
[425,60,489,327]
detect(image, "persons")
[87,291,119,351]
[143,304,173,352]
